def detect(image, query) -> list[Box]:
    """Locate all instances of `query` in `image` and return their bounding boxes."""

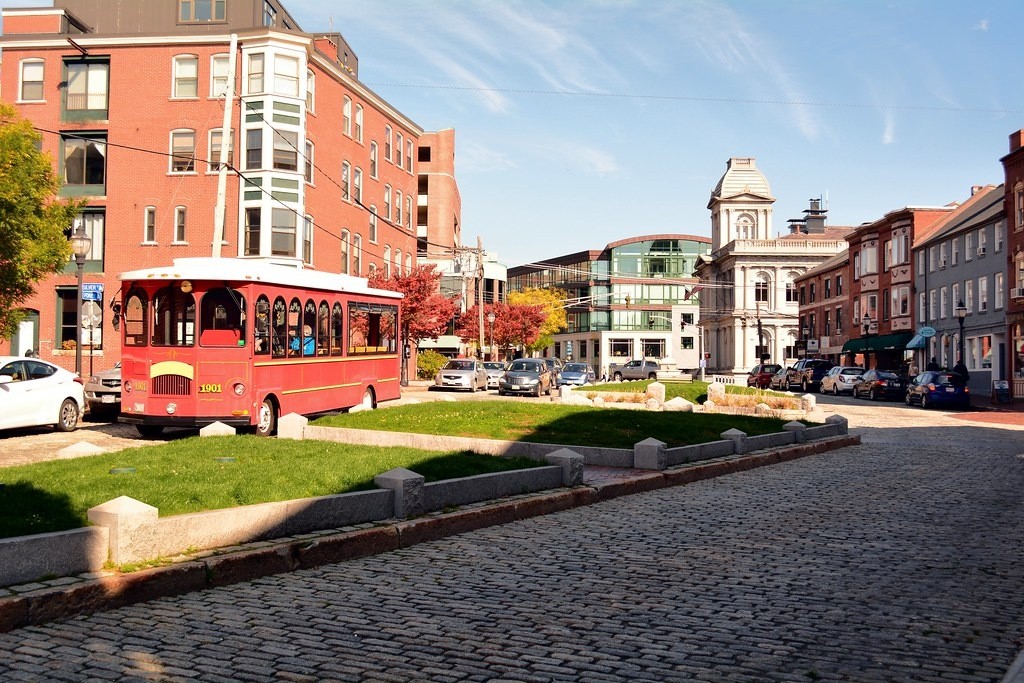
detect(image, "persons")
[907,360,918,384]
[953,360,970,389]
[899,361,908,378]
[927,357,939,371]
[255,322,323,355]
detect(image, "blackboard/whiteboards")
[992,380,1012,404]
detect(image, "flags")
[685,289,691,300]
[691,280,704,295]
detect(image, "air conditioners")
[852,318,858,325]
[977,246,986,255]
[1011,288,1024,299]
[938,259,947,269]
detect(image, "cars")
[484,361,509,388]
[84,361,121,422]
[820,366,866,396]
[0,356,84,432]
[612,359,661,381]
[905,370,969,409]
[746,363,787,390]
[435,359,488,393]
[543,357,595,390]
[499,358,552,397]
[853,369,902,401]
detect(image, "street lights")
[487,311,495,361]
[861,311,872,370]
[69,220,92,379]
[955,298,967,363]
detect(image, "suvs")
[785,357,837,392]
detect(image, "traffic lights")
[681,321,686,332]
[625,296,630,309]
[649,319,654,330]
[705,353,711,359]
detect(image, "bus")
[110,257,405,437]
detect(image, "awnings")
[906,334,925,349]
[842,332,914,353]
